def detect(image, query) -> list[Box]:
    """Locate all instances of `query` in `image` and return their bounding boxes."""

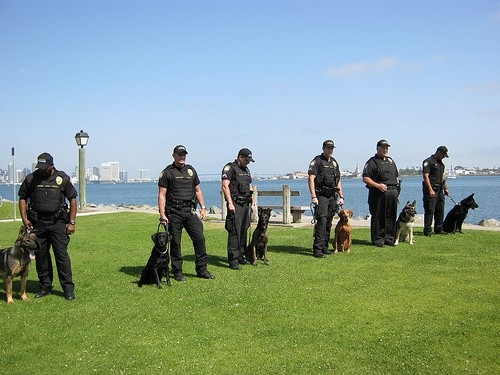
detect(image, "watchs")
[69,220,76,225]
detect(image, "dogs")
[136,232,172,289]
[443,193,479,234]
[333,210,353,254]
[394,200,415,246]
[245,206,272,265]
[0,226,37,304]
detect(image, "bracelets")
[201,205,206,209]
[340,197,344,200]
[312,196,316,199]
[444,188,447,190]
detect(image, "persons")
[221,148,255,270]
[158,145,215,281]
[422,146,449,236]
[363,140,401,247]
[308,140,344,257]
[18,153,78,300]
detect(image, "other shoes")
[435,231,447,234]
[385,240,394,245]
[425,232,431,237]
[376,241,384,247]
[323,248,331,255]
[313,252,325,257]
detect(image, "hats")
[437,146,449,158]
[323,140,336,148]
[35,153,53,170]
[174,145,188,154]
[239,148,255,162]
[377,139,391,147]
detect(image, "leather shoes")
[229,263,242,270]
[173,272,184,280]
[64,291,75,300]
[197,271,214,279]
[240,259,250,264]
[34,290,51,297]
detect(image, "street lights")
[74,130,89,209]
